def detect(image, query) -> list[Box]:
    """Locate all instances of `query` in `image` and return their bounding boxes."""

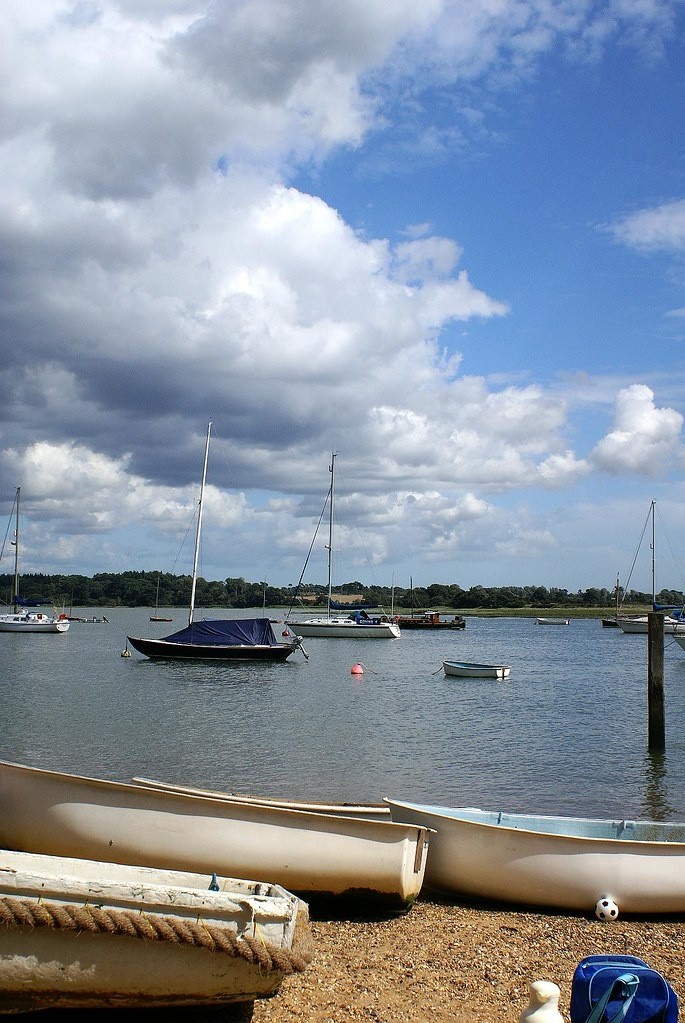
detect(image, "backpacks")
[569,954,678,1023]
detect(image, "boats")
[0,847,316,1015]
[441,659,513,679]
[536,617,572,627]
[0,756,439,919]
[670,632,685,651]
[377,791,684,919]
[370,569,469,629]
[79,614,111,623]
[126,774,483,821]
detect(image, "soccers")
[595,897,620,921]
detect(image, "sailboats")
[601,498,685,633]
[119,417,310,667]
[602,571,628,627]
[147,573,173,623]
[0,485,71,632]
[278,448,402,637]
